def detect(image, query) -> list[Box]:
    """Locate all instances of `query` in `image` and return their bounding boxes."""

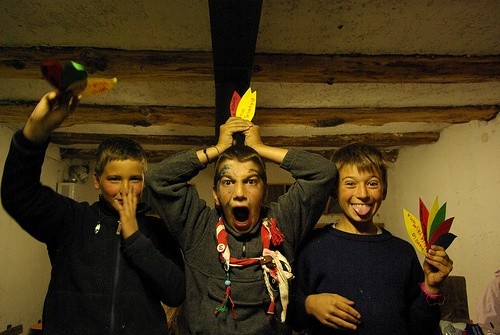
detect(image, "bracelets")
[421,281,441,298]
[203,148,210,163]
[214,146,220,157]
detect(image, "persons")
[285,142,453,335]
[142,117,338,335]
[0,90,186,335]
